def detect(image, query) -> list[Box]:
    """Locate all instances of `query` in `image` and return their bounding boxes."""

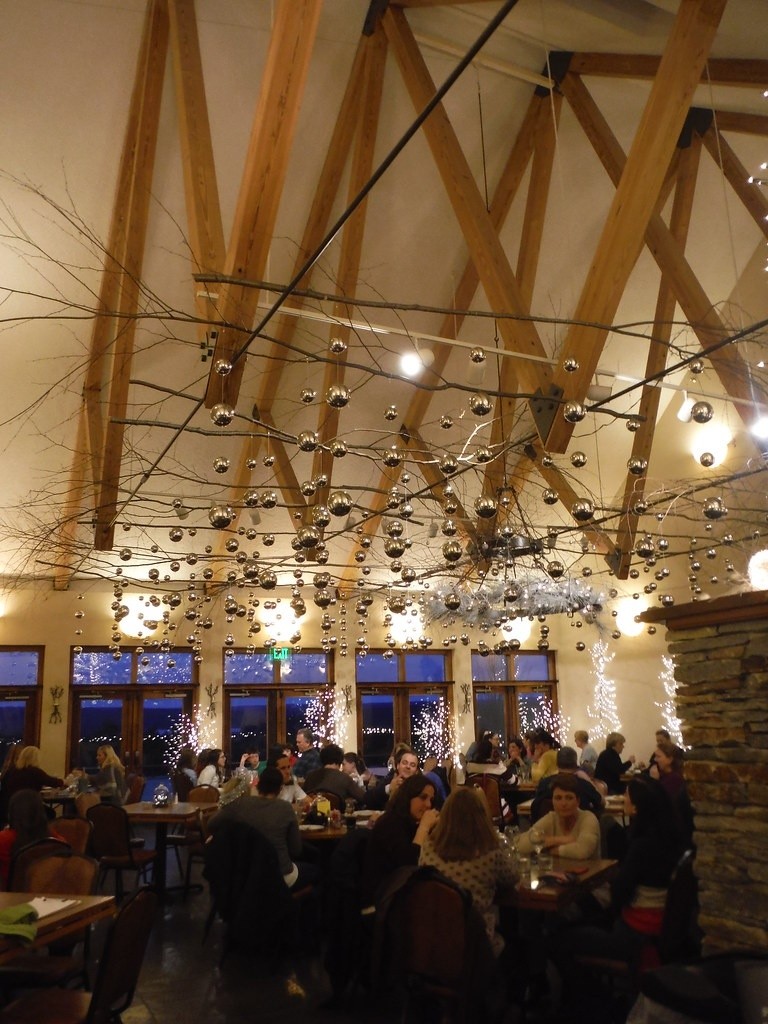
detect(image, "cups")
[316,801,331,826]
[517,847,531,884]
[345,799,355,816]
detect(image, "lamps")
[677,392,698,423]
[587,374,613,402]
[401,339,435,374]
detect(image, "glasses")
[492,737,500,741]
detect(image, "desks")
[516,795,624,816]
[499,783,536,793]
[492,854,620,1024]
[299,824,357,880]
[39,792,112,813]
[120,800,220,907]
[0,893,117,991]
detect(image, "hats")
[549,772,581,795]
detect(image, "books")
[28,897,81,920]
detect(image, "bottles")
[77,767,87,794]
[624,786,633,815]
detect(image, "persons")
[341,752,377,791]
[362,726,741,1024]
[0,789,67,896]
[0,744,69,833]
[302,744,367,813]
[207,768,321,933]
[78,745,130,806]
[175,729,323,806]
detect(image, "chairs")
[378,864,486,1024]
[626,847,699,1001]
[464,773,515,833]
[0,772,346,1024]
